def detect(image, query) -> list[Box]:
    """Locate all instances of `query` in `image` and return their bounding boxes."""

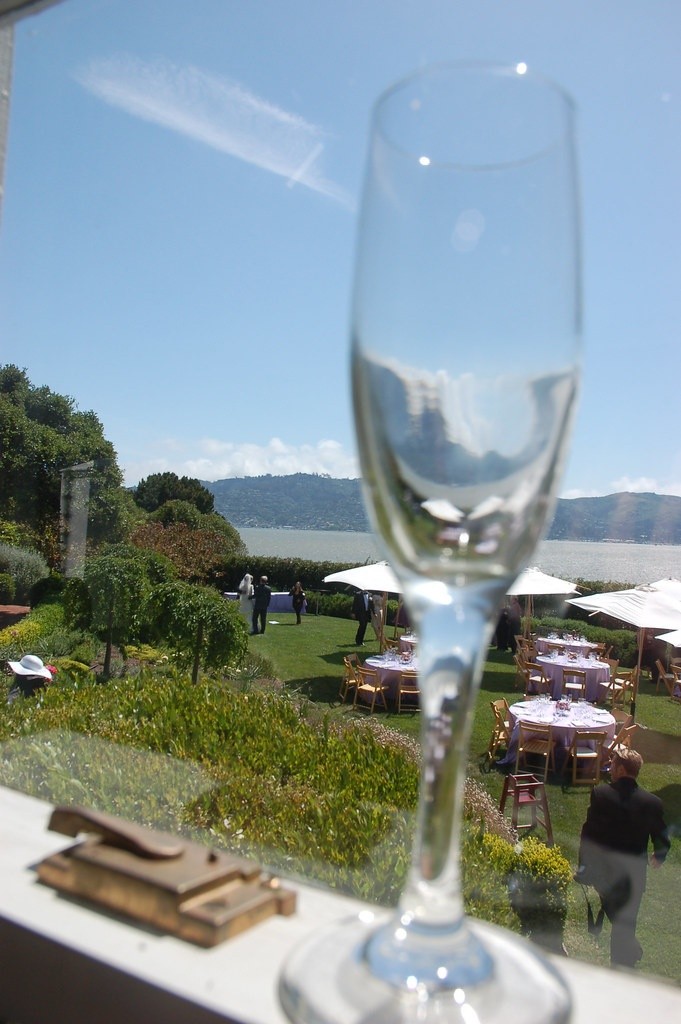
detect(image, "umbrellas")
[321,560,404,633]
[649,576,681,599]
[565,582,681,720]
[655,629,681,649]
[506,566,582,641]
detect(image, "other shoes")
[259,631,264,634]
[253,631,259,634]
[356,643,366,647]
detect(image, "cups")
[382,648,416,665]
[531,693,593,720]
[548,632,586,643]
[550,648,597,664]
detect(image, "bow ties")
[364,593,368,595]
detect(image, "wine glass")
[280,60,580,1022]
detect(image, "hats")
[8,655,51,679]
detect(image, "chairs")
[339,607,680,842]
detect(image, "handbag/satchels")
[573,845,604,886]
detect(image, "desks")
[360,654,418,705]
[223,592,308,613]
[532,653,612,702]
[496,698,615,774]
[400,635,417,651]
[533,638,593,663]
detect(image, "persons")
[237,573,255,632]
[288,581,306,625]
[370,591,382,641]
[644,626,667,684]
[497,594,524,658]
[352,588,378,646]
[249,575,271,633]
[7,655,52,698]
[576,746,670,968]
